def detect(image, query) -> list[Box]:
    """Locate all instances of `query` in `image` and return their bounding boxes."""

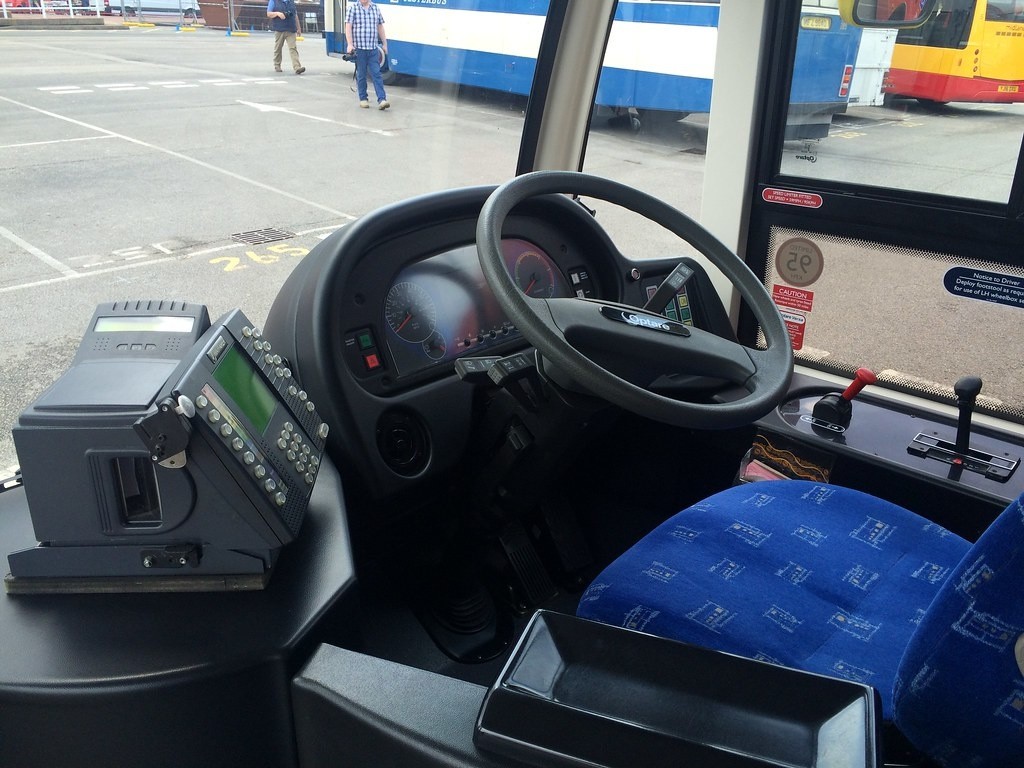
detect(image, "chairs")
[578,478,1024,768]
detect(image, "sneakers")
[378,100,389,110]
[361,100,367,107]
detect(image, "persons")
[344,0,390,110]
[267,0,305,74]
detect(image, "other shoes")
[274,68,280,71]
[295,67,303,73]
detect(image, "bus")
[324,0,1024,140]
